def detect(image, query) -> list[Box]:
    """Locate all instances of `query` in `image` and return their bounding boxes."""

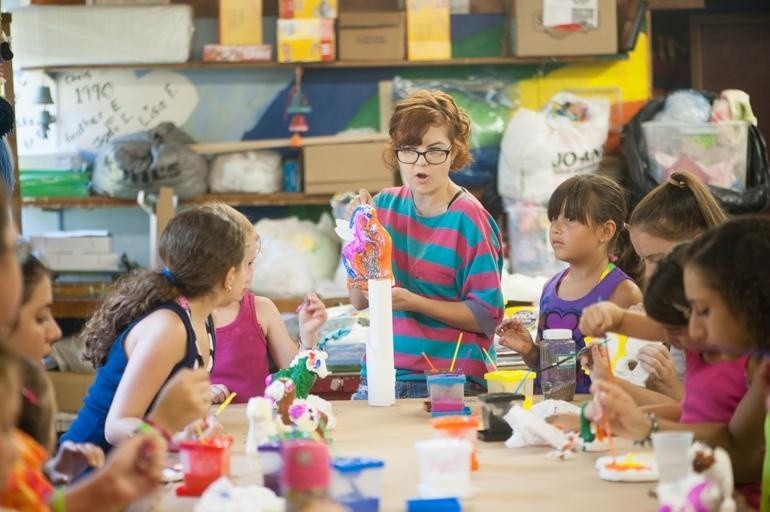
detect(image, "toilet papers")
[367,276,397,406]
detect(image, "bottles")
[539,328,577,402]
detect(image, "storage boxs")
[639,121,749,193]
[303,142,397,196]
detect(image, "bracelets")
[296,336,321,353]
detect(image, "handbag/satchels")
[498,91,610,206]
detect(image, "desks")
[211,398,655,512]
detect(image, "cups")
[651,429,694,482]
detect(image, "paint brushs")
[205,392,237,423]
[443,349,472,400]
[536,338,611,373]
[422,352,439,372]
[599,390,617,464]
[296,300,309,313]
[449,333,463,373]
[515,368,532,394]
[482,347,498,372]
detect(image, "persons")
[342,85,505,399]
[210,230,331,407]
[0,178,171,512]
[541,168,770,511]
[56,204,249,485]
[497,174,643,396]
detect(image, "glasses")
[394,145,452,164]
